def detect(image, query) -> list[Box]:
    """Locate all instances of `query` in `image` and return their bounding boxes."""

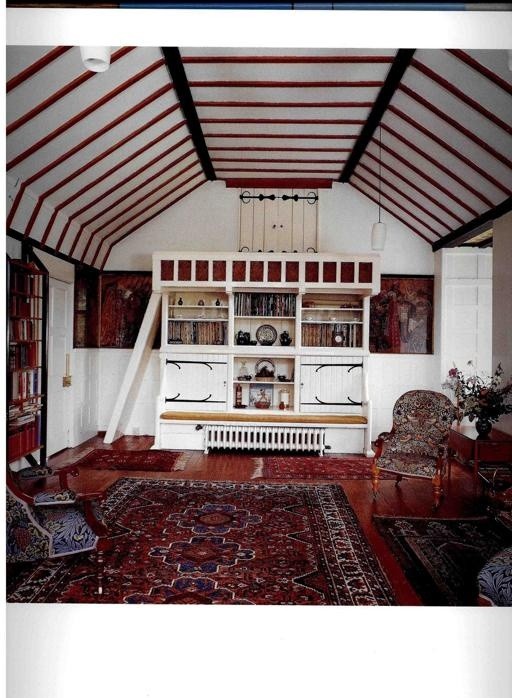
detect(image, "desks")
[443,423,512,499]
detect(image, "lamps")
[372,125,387,251]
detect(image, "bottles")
[280,386,290,409]
[235,384,242,406]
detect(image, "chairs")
[370,388,458,508]
[5,463,113,597]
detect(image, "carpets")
[7,476,404,605]
[62,449,190,473]
[371,511,508,606]
[255,453,401,481]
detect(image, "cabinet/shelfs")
[151,251,381,459]
[5,249,48,477]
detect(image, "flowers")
[441,359,511,423]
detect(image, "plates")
[255,324,278,345]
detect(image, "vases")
[475,419,492,440]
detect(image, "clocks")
[332,330,346,345]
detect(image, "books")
[167,319,228,346]
[301,322,364,347]
[234,292,297,316]
[7,262,43,430]
